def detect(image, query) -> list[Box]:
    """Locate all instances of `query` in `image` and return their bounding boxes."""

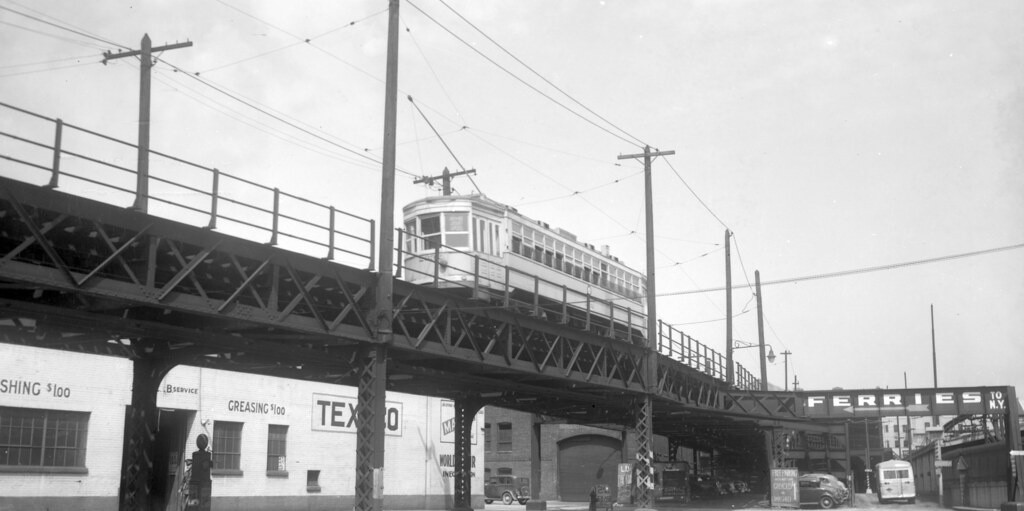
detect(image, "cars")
[800,475,848,509]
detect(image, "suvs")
[484,475,529,506]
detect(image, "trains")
[403,194,651,344]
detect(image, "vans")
[875,459,915,504]
